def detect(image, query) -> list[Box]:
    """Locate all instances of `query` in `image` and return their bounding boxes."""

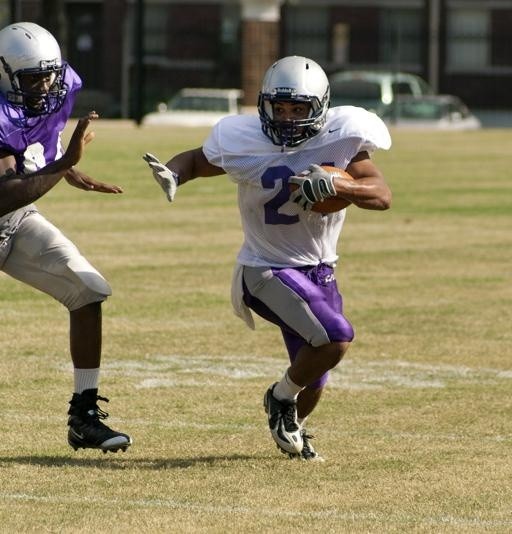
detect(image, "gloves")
[289,162,342,211]
[142,151,178,203]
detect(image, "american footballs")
[289,166,355,213]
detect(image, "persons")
[143,55,392,463]
[1,21,133,454]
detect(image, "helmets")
[0,21,69,117]
[256,54,332,147]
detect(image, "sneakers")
[67,416,131,453]
[265,381,305,453]
[290,430,321,464]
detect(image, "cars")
[326,71,436,116]
[368,93,483,132]
[140,87,246,129]
